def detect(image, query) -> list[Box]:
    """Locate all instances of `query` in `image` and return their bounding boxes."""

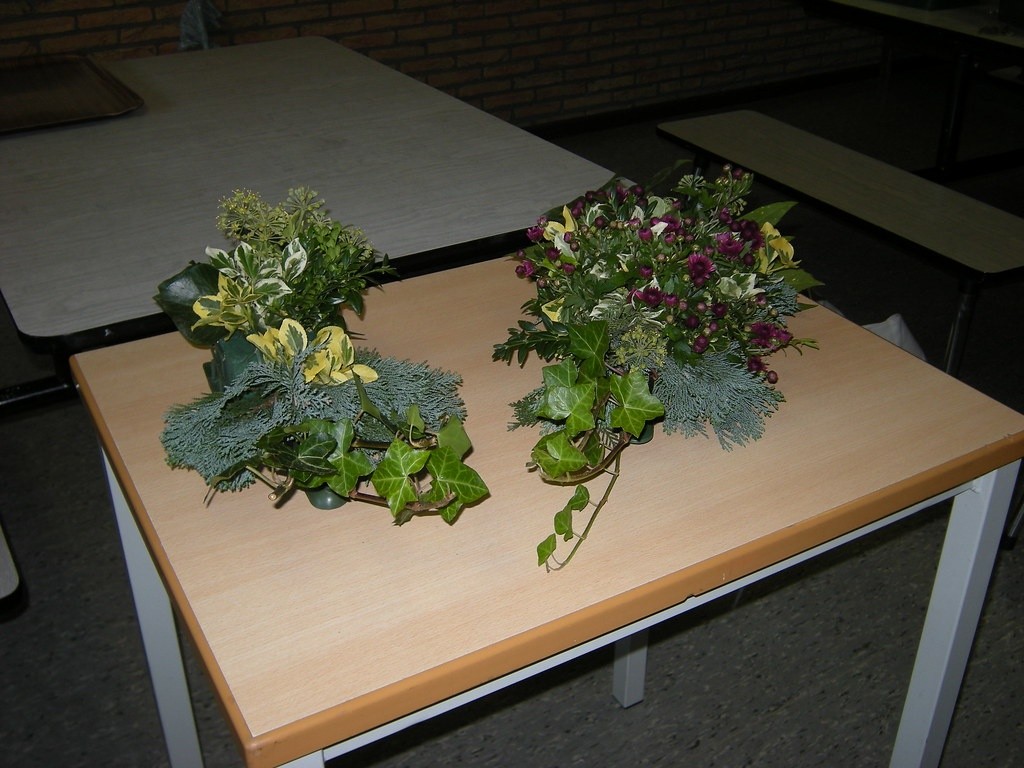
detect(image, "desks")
[803,0,1024,185]
[0,38,636,349]
[68,254,1024,768]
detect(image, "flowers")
[488,159,826,571]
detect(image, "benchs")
[656,108,1023,378]
